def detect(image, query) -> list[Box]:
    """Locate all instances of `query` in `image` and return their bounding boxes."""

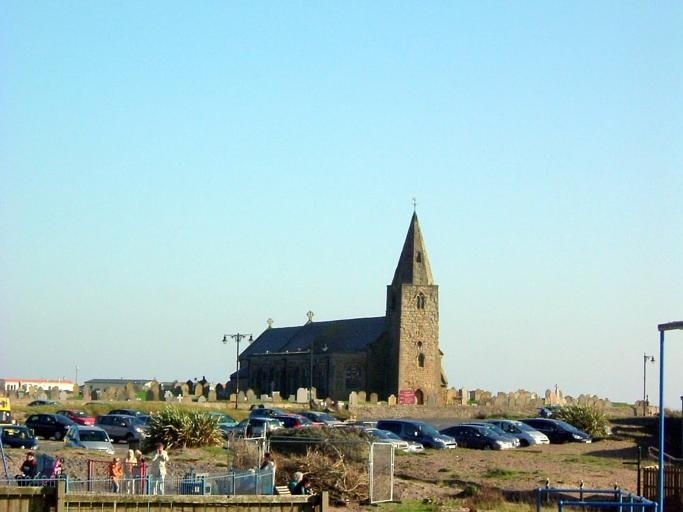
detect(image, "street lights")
[72,365,80,384]
[657,318,681,512]
[642,353,655,417]
[298,338,330,411]
[221,332,253,410]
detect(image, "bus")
[0,394,13,434]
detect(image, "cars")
[0,425,41,452]
[25,400,58,408]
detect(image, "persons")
[14,451,38,486]
[138,457,148,496]
[48,456,64,482]
[122,449,137,494]
[288,469,303,495]
[292,474,314,511]
[133,449,142,465]
[259,450,272,469]
[108,455,121,492]
[150,440,168,496]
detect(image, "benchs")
[274,485,293,495]
